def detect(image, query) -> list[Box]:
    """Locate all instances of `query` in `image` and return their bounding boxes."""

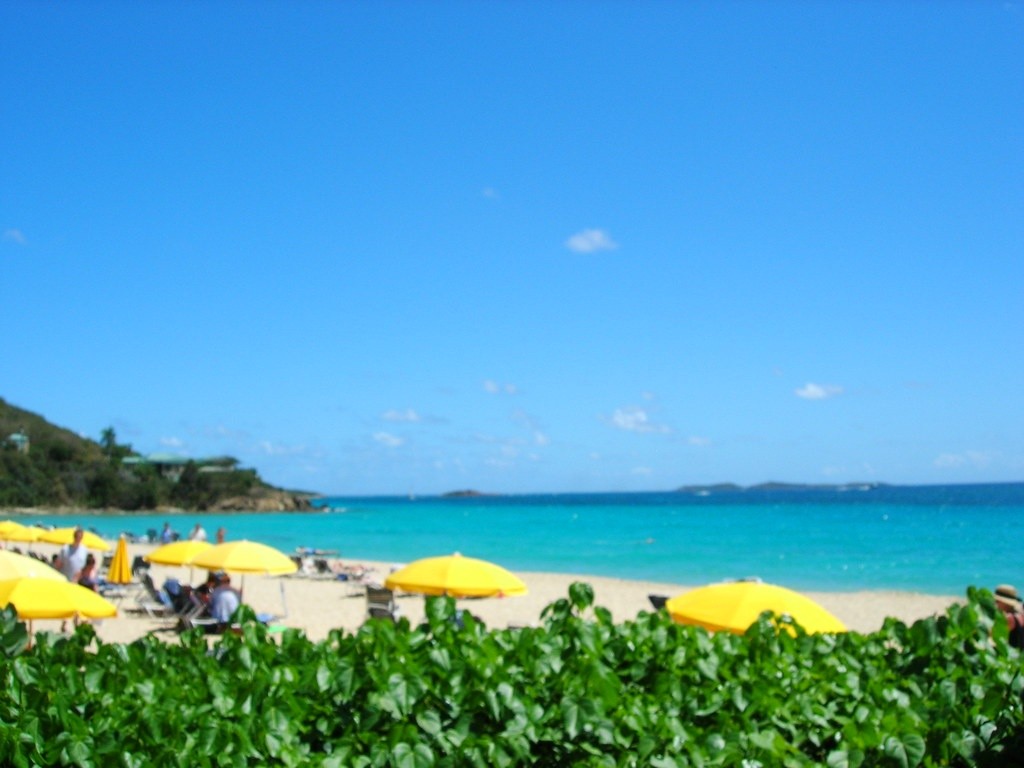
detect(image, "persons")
[291,555,364,576]
[993,585,1024,650]
[12,522,243,633]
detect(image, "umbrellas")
[0,519,112,553]
[0,550,117,650]
[108,534,132,592]
[386,552,529,600]
[143,540,214,585]
[666,581,848,638]
[193,539,298,603]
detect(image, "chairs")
[365,584,399,625]
[133,571,219,633]
